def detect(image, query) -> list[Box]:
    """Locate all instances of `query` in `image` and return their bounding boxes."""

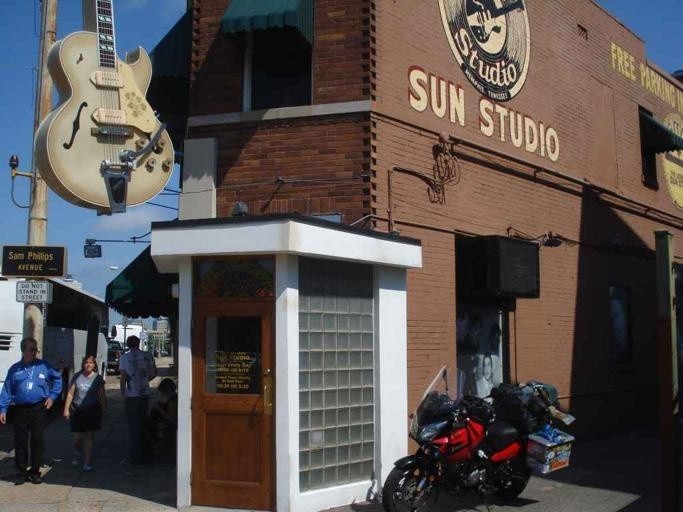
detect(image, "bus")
[0,270,108,406]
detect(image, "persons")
[478,355,495,397]
[0,339,63,485]
[119,335,158,465]
[63,355,107,471]
[147,378,177,427]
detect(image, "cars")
[108,340,125,374]
[148,348,168,357]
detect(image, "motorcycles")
[383,365,560,512]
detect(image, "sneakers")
[14,477,23,485]
[34,474,41,483]
[72,452,81,465]
[84,464,91,471]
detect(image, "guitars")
[465,0,524,42]
[34,0,175,214]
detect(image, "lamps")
[512,230,562,248]
